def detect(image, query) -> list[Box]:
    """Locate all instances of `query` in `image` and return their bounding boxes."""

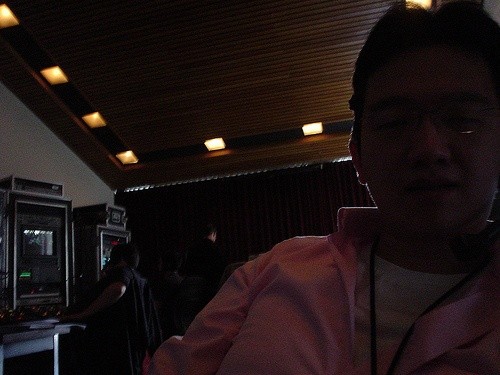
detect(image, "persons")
[142,0,500,375]
[160,223,232,341]
[155,247,185,284]
[58,244,146,321]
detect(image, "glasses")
[357,91,500,140]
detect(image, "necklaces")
[369,232,492,375]
